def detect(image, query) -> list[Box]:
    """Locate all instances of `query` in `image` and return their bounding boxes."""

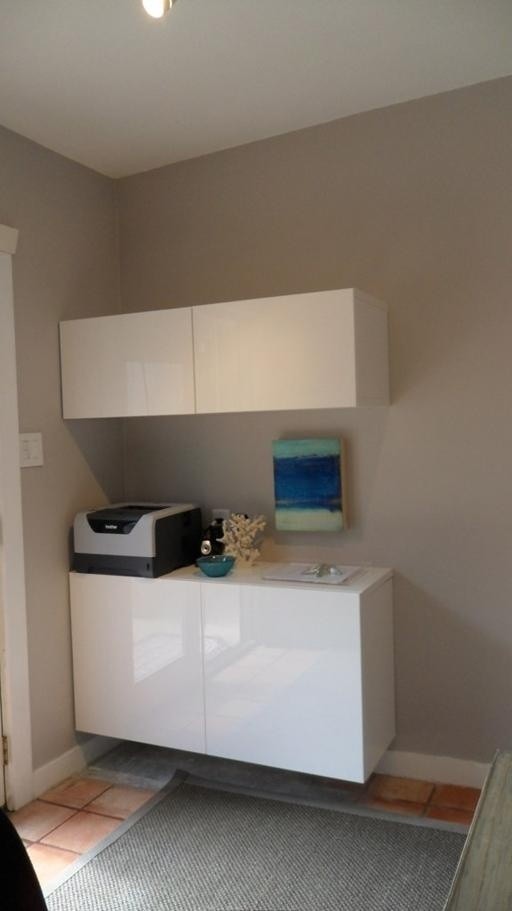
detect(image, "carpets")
[44,767,467,911]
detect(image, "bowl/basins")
[196,550,235,580]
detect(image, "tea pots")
[199,516,230,568]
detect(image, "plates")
[261,556,366,587]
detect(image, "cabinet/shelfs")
[59,287,392,421]
[69,559,398,787]
